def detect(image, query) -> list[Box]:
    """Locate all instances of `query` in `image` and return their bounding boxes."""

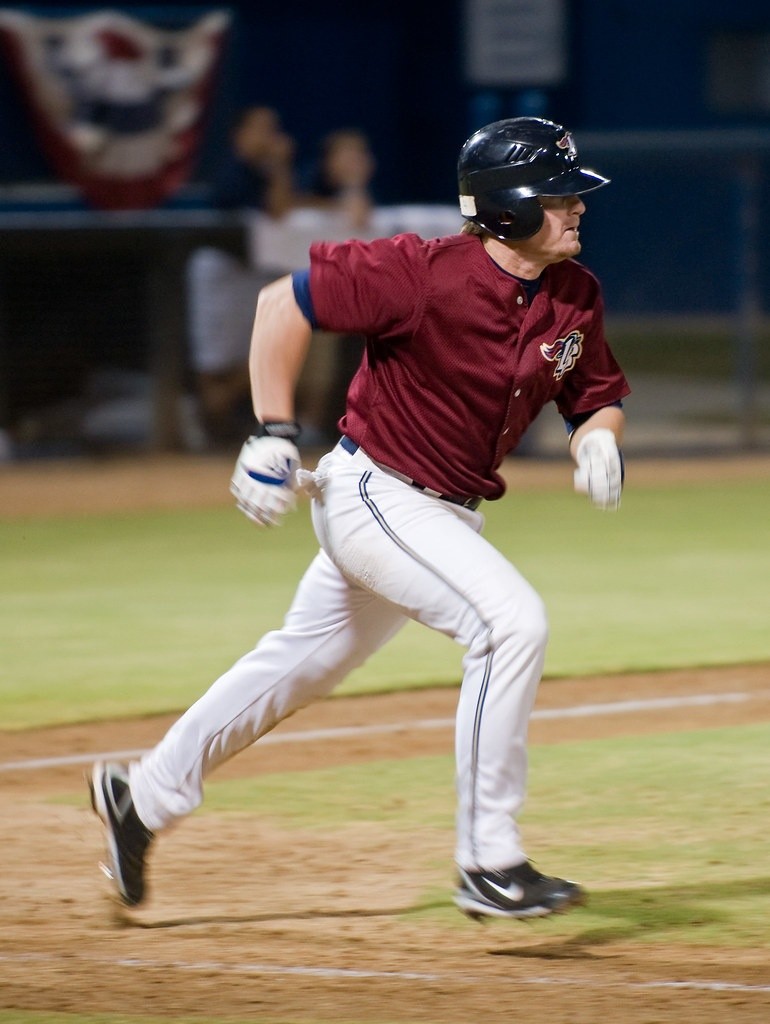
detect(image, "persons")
[83,117,631,921]
[181,107,380,455]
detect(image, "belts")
[339,435,484,513]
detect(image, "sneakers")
[86,757,147,907]
[451,860,588,917]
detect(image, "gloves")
[229,418,302,527]
[572,429,625,512]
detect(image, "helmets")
[453,116,610,243]
